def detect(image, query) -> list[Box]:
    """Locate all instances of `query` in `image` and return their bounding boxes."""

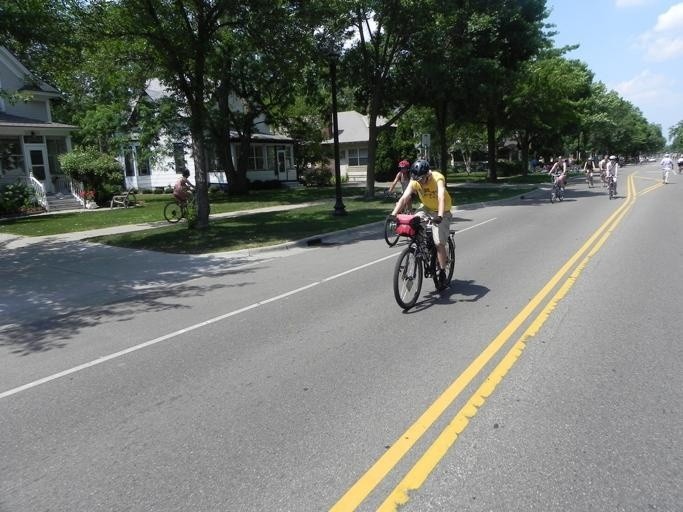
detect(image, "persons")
[387,160,418,214]
[660,153,683,184]
[173,169,195,218]
[386,159,452,288]
[531,155,625,195]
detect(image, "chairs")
[110,187,139,209]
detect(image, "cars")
[640,159,648,165]
[648,157,656,162]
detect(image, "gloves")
[431,216,444,226]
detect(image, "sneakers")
[437,268,447,286]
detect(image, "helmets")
[398,161,410,169]
[410,159,431,179]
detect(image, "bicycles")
[383,213,458,310]
[163,186,210,224]
[384,191,417,247]
[531,164,616,204]
